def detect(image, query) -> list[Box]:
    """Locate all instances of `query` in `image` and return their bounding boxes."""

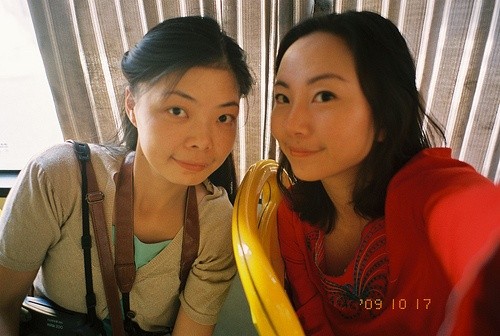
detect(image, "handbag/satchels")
[17,298,106,336]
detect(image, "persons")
[271,9,500,336]
[0,15,255,336]
